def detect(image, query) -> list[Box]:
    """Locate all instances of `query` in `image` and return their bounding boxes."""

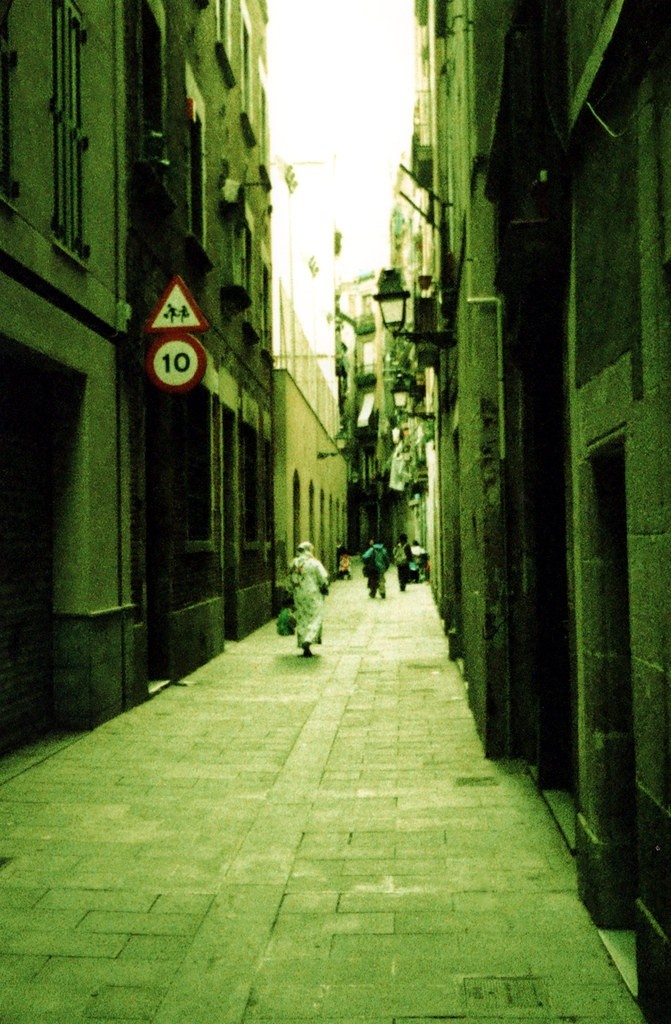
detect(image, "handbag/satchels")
[275,608,296,636]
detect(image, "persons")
[337,538,353,580]
[409,541,426,582]
[362,539,389,598]
[393,533,412,591]
[290,542,329,656]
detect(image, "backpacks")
[394,543,409,565]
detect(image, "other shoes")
[302,643,313,657]
